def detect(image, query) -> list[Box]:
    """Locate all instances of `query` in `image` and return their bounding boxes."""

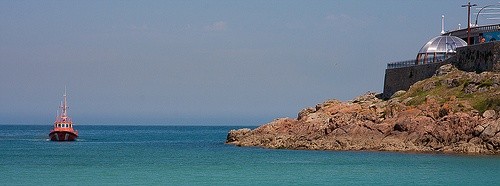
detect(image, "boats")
[48,84,78,141]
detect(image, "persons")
[489,37,497,41]
[479,37,486,43]
